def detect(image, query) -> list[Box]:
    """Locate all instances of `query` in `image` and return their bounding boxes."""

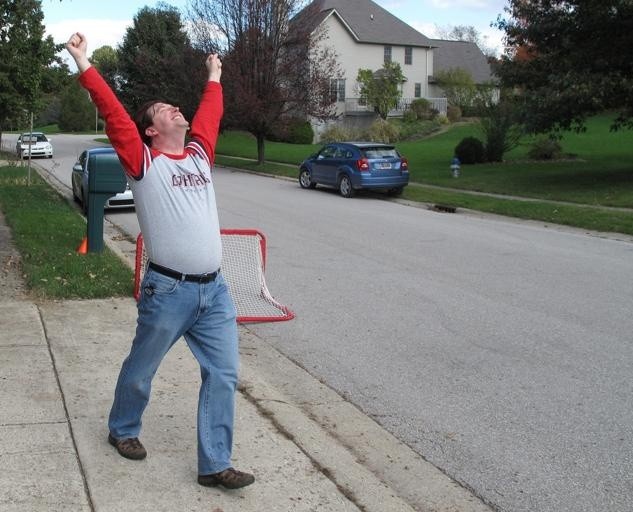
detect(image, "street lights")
[23,109,33,186]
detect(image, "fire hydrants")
[450,158,461,181]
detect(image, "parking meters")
[83,153,127,253]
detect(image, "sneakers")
[198,467,254,488]
[109,432,147,459]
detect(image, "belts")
[147,259,220,283]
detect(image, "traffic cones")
[77,235,88,254]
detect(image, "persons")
[66,31,255,488]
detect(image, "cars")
[298,141,409,198]
[17,132,53,159]
[72,147,136,210]
